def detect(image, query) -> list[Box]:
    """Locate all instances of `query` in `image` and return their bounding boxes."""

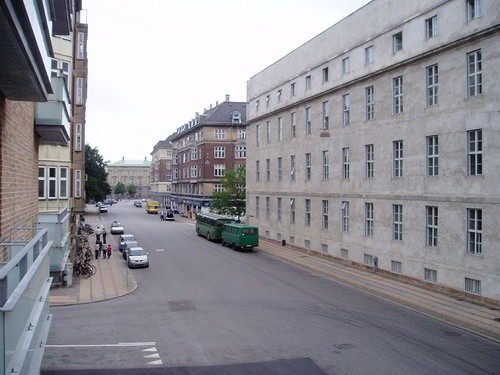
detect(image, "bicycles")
[73,235,97,279]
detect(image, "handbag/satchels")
[105,232,107,235]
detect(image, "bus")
[196,213,235,241]
[146,201,159,214]
[222,223,259,252]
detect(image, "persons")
[102,241,108,259]
[101,228,107,244]
[96,226,102,240]
[95,241,100,259]
[96,238,102,254]
[159,210,164,221]
[107,244,111,258]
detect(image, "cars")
[118,234,139,261]
[134,199,146,207]
[96,201,108,213]
[111,221,125,235]
[127,247,150,269]
